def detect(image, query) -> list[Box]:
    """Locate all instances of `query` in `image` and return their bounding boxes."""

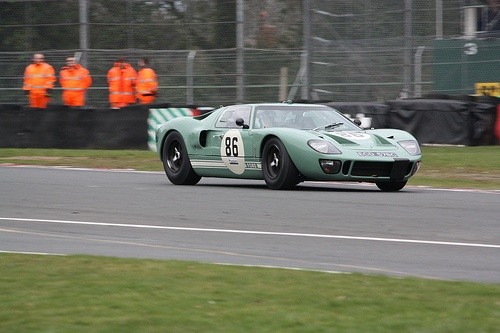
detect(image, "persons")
[132,57,159,105]
[58,56,92,106]
[23,53,57,109]
[106,54,137,108]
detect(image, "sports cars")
[154,101,421,190]
